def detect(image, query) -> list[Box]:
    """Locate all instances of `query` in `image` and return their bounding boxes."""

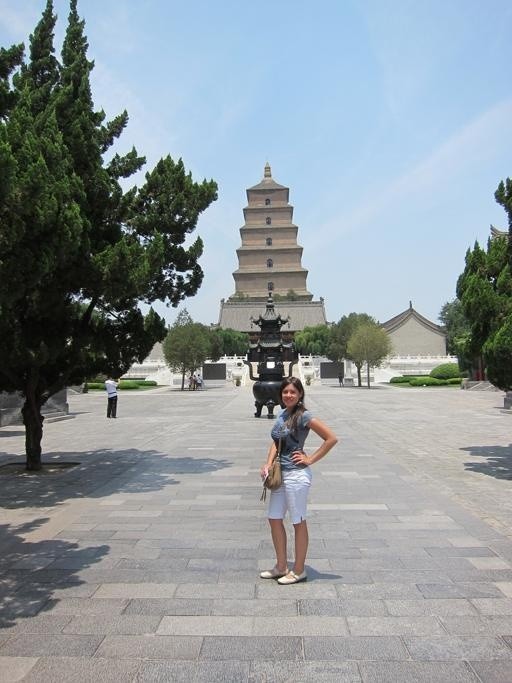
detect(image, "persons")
[188,373,203,390]
[258,375,336,586]
[104,373,120,420]
[337,372,344,386]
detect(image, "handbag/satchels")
[260,455,283,489]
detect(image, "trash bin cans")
[504,398,511,408]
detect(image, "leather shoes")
[276,569,307,585]
[258,566,290,579]
